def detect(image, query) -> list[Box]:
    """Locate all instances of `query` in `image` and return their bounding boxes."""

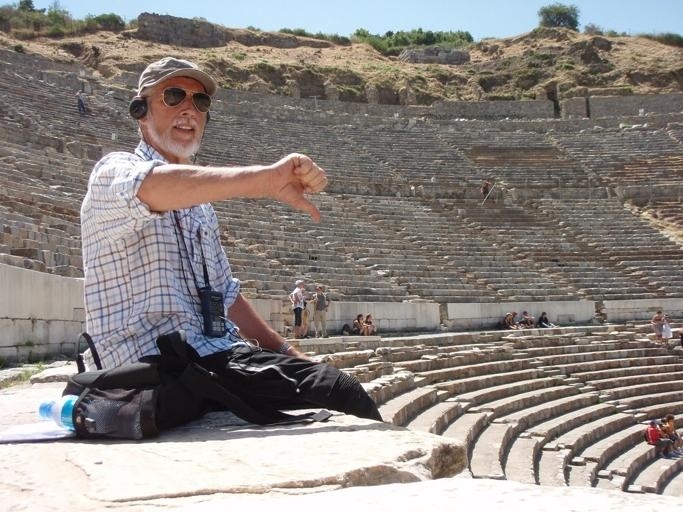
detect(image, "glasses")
[161,86,212,113]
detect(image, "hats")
[137,56,218,98]
[294,279,305,285]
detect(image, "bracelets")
[280,341,293,353]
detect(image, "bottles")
[38,394,143,439]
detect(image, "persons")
[289,279,331,339]
[644,414,683,460]
[500,311,560,330]
[481,179,493,199]
[75,90,86,113]
[79,57,386,424]
[662,313,673,345]
[343,313,377,336]
[651,310,662,343]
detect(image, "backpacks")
[61,329,333,439]
